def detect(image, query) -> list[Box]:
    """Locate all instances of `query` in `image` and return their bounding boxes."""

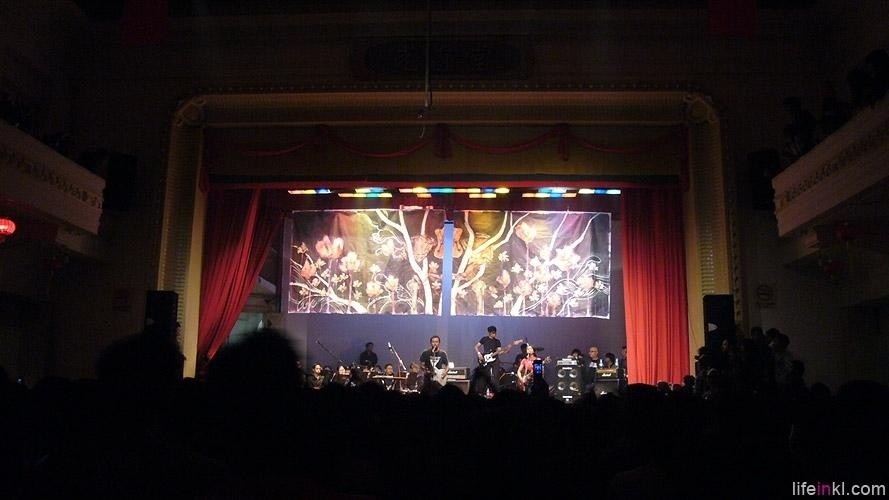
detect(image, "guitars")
[478,351,498,366]
[410,362,448,387]
[516,356,551,390]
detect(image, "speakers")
[144,290,178,339]
[555,364,582,404]
[703,294,735,346]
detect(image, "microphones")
[432,345,436,352]
[387,341,392,351]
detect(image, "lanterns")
[0,217,16,243]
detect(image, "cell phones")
[533,363,542,378]
